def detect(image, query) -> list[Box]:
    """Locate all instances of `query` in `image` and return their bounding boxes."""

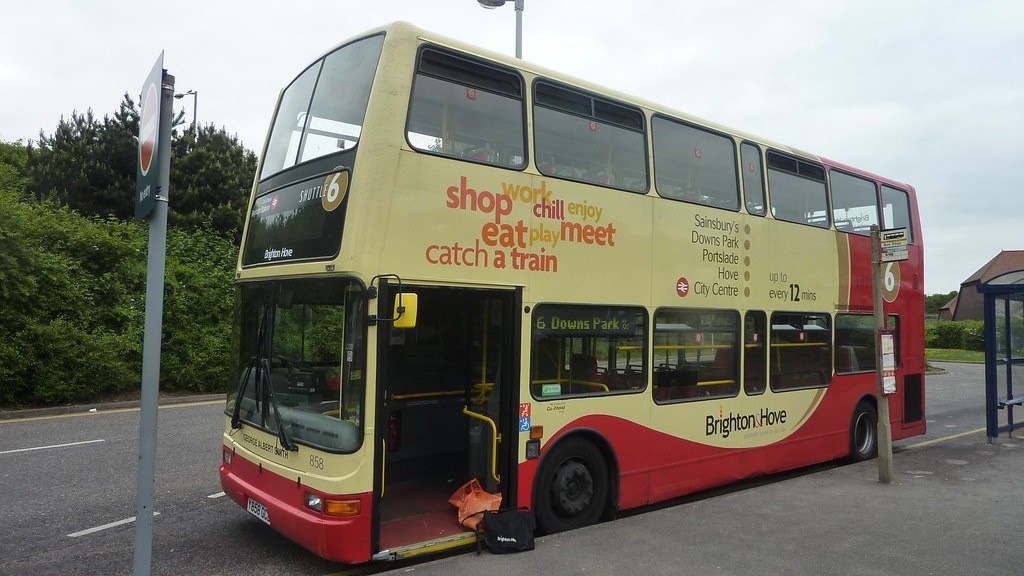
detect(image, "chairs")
[561,359,781,400]
[463,147,879,236]
[824,346,859,372]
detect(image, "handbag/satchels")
[475,509,537,554]
[448,479,502,533]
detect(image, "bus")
[216,18,929,569]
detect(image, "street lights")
[477,0,525,59]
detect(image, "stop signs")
[134,49,165,224]
